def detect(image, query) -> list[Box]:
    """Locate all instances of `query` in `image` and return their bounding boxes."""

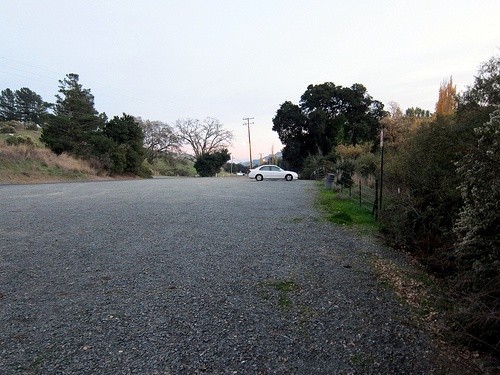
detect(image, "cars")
[246,164,299,181]
